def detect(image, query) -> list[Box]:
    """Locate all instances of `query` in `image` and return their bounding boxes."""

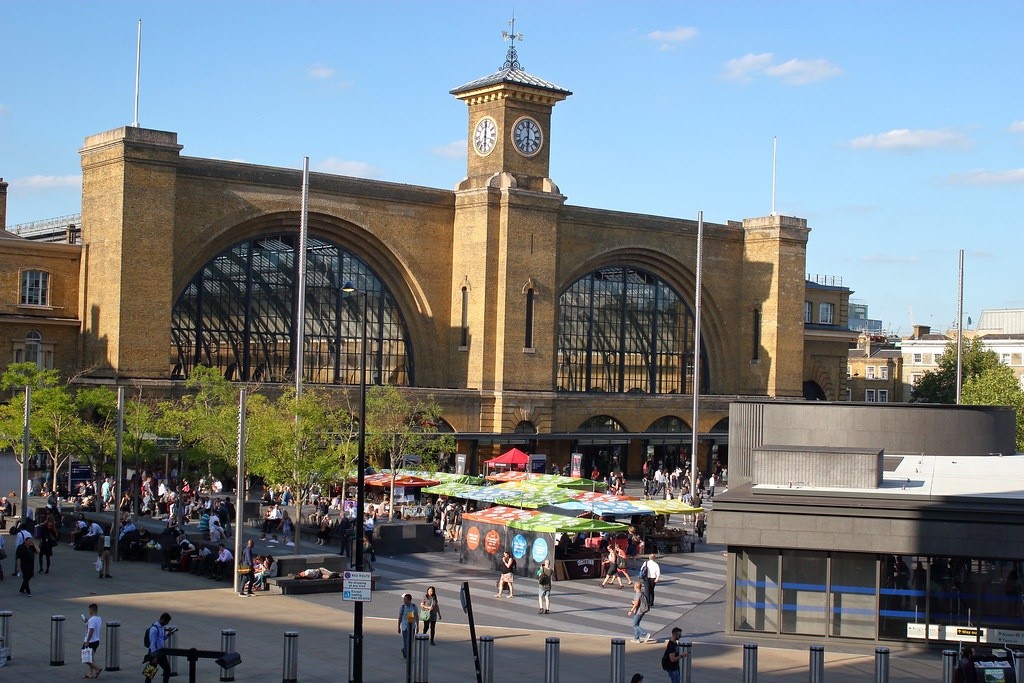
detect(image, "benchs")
[266,574,381,594]
[274,524,351,548]
[58,528,160,563]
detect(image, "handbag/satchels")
[96,555,103,573]
[238,565,252,574]
[618,554,626,569]
[640,562,648,579]
[537,567,549,585]
[419,611,431,621]
[142,663,158,680]
[81,646,93,663]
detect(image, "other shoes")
[538,608,549,614]
[239,593,247,597]
[38,569,43,573]
[247,592,255,596]
[431,642,436,646]
[105,575,112,578]
[401,649,406,658]
[44,570,49,574]
[12,572,19,577]
[630,633,651,643]
[494,594,514,598]
[19,591,32,597]
[599,582,632,589]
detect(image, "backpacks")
[614,551,621,565]
[144,623,159,648]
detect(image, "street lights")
[342,280,369,683]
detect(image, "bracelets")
[681,655,683,658]
[630,610,633,612]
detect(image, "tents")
[348,470,705,580]
[485,449,530,475]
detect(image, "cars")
[59,462,92,498]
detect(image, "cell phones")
[171,628,176,632]
[81,614,86,622]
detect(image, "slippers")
[82,675,92,679]
[95,668,102,678]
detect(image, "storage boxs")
[992,649,1007,657]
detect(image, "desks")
[973,650,1015,683]
[645,534,694,553]
[564,560,601,579]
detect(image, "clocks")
[474,119,496,154]
[514,120,541,154]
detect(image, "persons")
[0,465,237,599]
[420,586,442,645]
[82,604,102,679]
[537,560,556,614]
[239,540,279,597]
[258,477,478,571]
[955,645,976,683]
[640,554,660,606]
[495,551,514,598]
[145,612,175,683]
[667,628,689,683]
[549,456,727,589]
[889,556,1024,619]
[628,582,651,643]
[397,594,419,658]
[631,673,644,683]
